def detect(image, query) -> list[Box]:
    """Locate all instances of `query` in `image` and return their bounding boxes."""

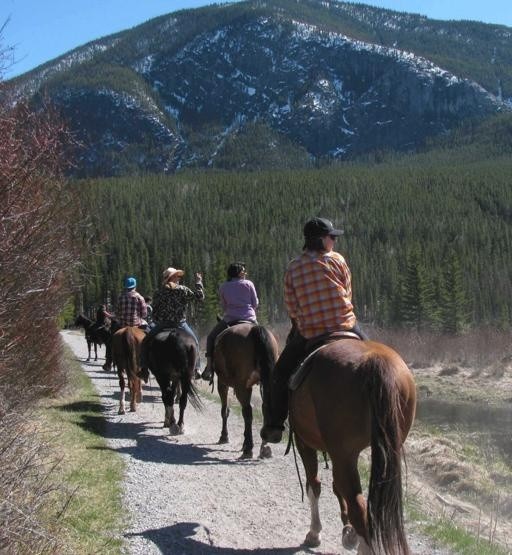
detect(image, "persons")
[102,277,149,376]
[202,261,261,382]
[139,295,154,330]
[89,305,115,340]
[260,215,370,447]
[135,266,206,384]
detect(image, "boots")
[202,356,214,378]
[103,358,112,371]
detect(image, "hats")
[123,278,137,289]
[162,267,184,286]
[304,218,345,236]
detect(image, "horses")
[285,336,420,555]
[72,311,205,438]
[208,314,281,462]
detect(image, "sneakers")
[259,427,281,443]
[195,369,202,379]
[136,371,149,379]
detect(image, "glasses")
[330,235,335,240]
